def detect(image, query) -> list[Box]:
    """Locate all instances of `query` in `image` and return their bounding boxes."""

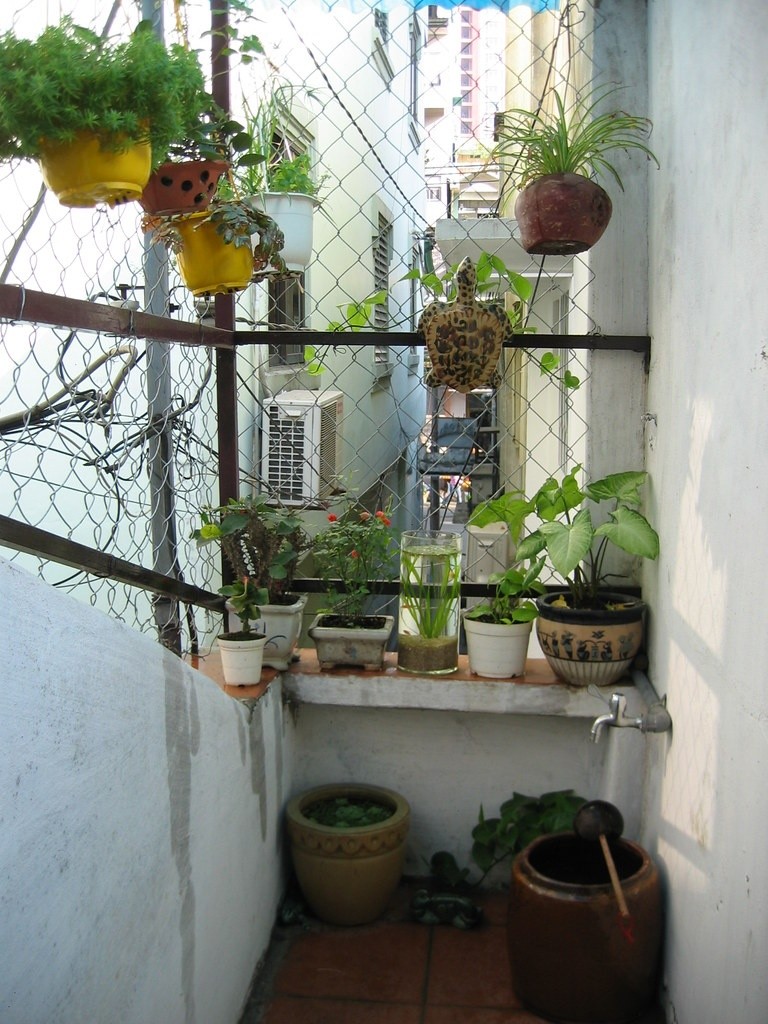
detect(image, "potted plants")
[482,85,661,256]
[286,780,412,929]
[0,0,331,297]
[518,463,659,685]
[462,486,547,678]
[215,574,271,687]
[190,493,308,672]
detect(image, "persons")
[442,475,461,508]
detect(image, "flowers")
[305,472,399,625]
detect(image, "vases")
[396,529,463,675]
[307,613,397,672]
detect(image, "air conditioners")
[263,389,345,509]
[464,525,518,607]
